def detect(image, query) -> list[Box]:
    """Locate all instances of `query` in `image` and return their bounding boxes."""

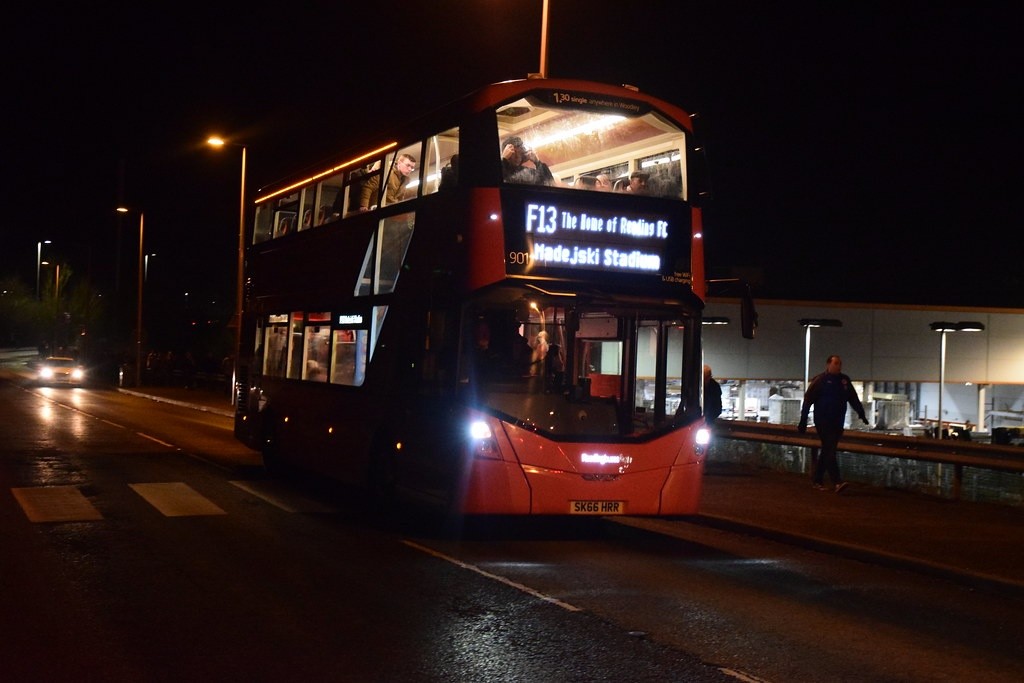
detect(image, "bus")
[232,73,711,540]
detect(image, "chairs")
[613,179,632,192]
[574,176,604,190]
[269,335,286,376]
[314,334,348,383]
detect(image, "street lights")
[698,317,730,417]
[206,134,247,405]
[37,240,52,309]
[929,321,985,495]
[797,319,844,474]
[116,206,143,385]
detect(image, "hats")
[630,171,650,179]
[502,136,523,153]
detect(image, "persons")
[335,136,651,212]
[798,356,869,494]
[704,365,723,467]
[259,323,566,395]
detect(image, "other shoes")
[834,481,848,493]
[813,483,828,490]
